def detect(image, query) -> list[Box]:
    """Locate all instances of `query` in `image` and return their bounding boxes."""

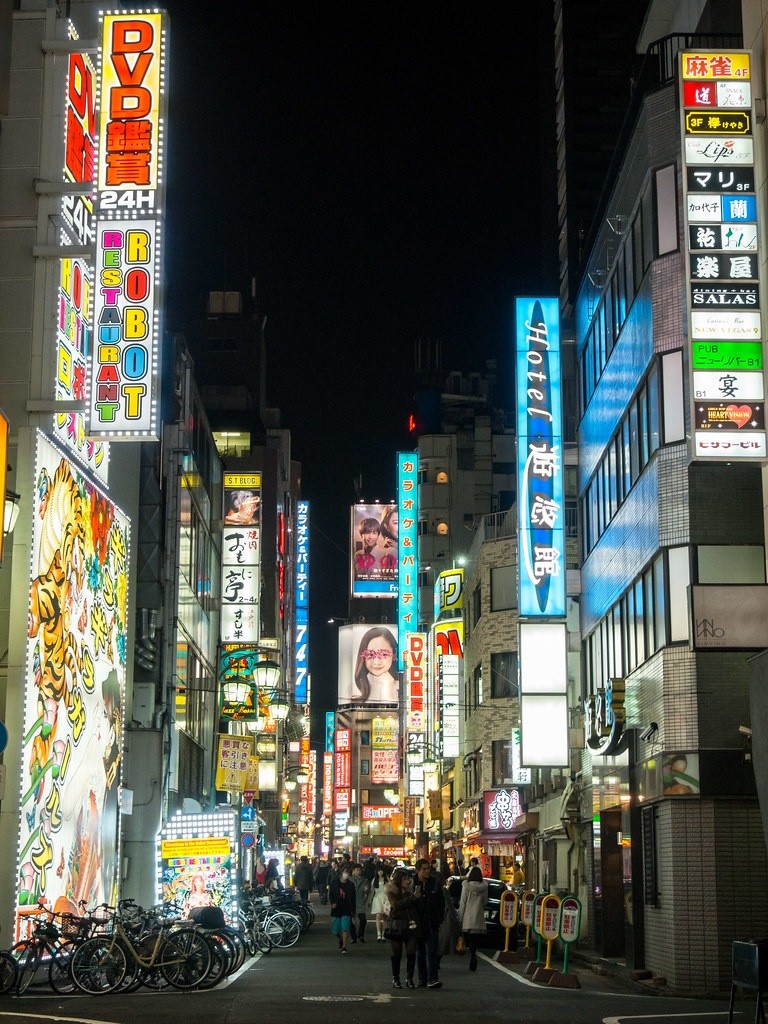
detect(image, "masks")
[378,871,384,877]
[342,873,349,880]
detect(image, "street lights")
[406,742,443,874]
[209,646,281,814]
[235,689,290,890]
[276,766,309,850]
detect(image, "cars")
[390,866,416,887]
[443,875,518,947]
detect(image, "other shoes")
[342,947,347,955]
[427,980,443,989]
[377,930,386,942]
[391,980,401,988]
[418,979,426,987]
[339,939,343,951]
[351,937,356,943]
[405,975,416,989]
[358,935,367,944]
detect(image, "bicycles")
[0,875,315,999]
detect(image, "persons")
[457,867,488,970]
[352,626,400,702]
[385,870,421,988]
[309,854,398,954]
[430,860,438,873]
[432,871,456,969]
[409,859,446,989]
[354,505,398,573]
[192,875,205,893]
[466,858,482,876]
[266,859,281,888]
[294,856,313,902]
[512,863,525,885]
[453,860,471,875]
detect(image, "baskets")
[62,913,86,936]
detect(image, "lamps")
[220,649,292,741]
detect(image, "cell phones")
[415,886,421,893]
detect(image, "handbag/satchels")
[456,934,466,952]
